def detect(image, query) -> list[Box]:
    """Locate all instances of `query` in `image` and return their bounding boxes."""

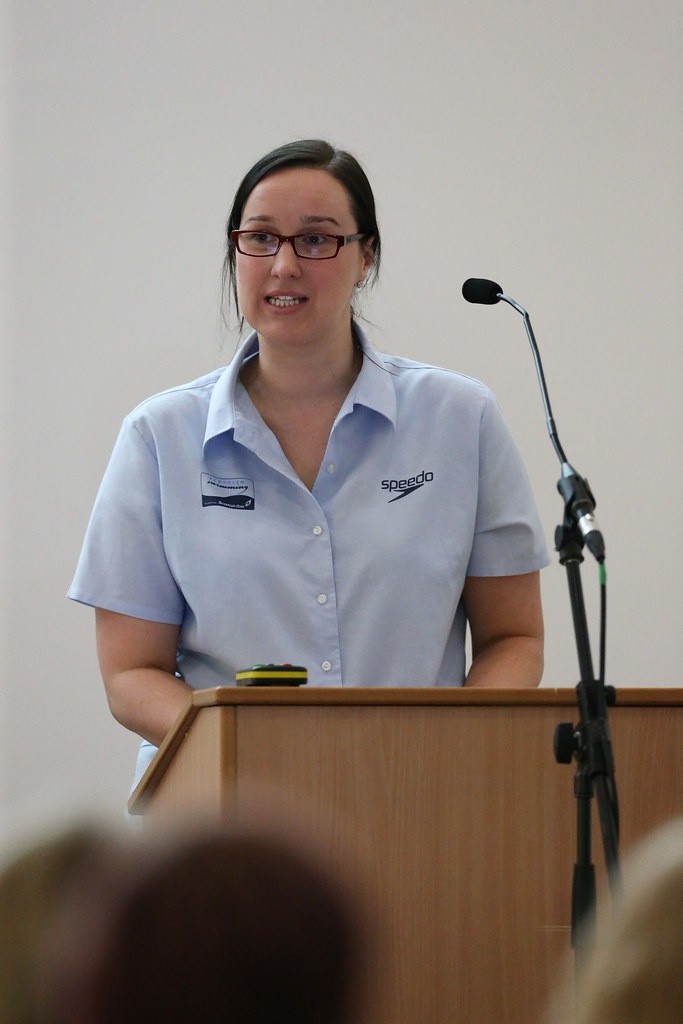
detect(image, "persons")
[545,806,683,1024]
[59,826,387,1024]
[65,134,552,814]
[1,829,123,1023]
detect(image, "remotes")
[234,664,307,687]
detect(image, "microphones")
[462,277,605,561]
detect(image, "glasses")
[231,229,364,260]
